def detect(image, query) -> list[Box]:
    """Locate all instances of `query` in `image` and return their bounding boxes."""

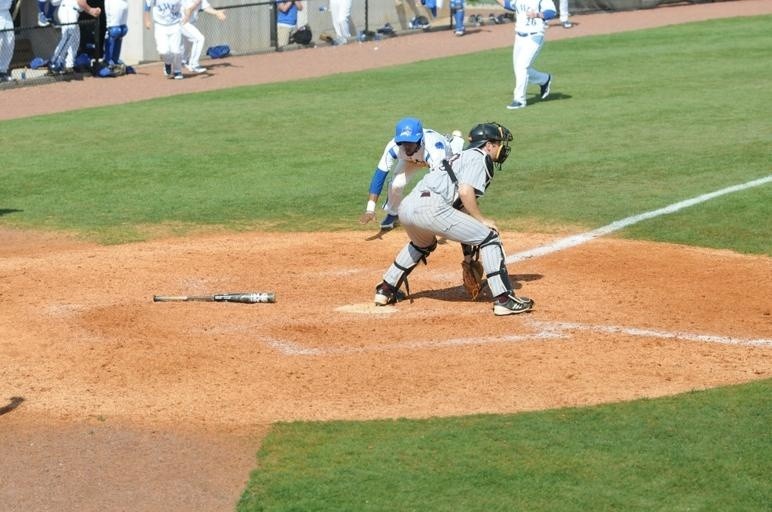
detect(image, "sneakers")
[456,31,464,35]
[38,14,61,30]
[380,214,399,229]
[165,61,206,79]
[494,299,534,316]
[540,74,551,99]
[564,21,572,28]
[507,101,527,109]
[374,287,404,305]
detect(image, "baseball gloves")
[462,260,483,300]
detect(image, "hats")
[395,118,423,144]
[30,57,49,69]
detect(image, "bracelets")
[366,200,376,213]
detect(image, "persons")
[497,1,552,107]
[362,117,456,231]
[422,0,571,36]
[373,123,533,320]
[277,1,358,52]
[2,0,226,79]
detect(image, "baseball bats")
[154,293,276,304]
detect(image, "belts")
[517,32,537,37]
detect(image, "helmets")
[462,122,513,164]
